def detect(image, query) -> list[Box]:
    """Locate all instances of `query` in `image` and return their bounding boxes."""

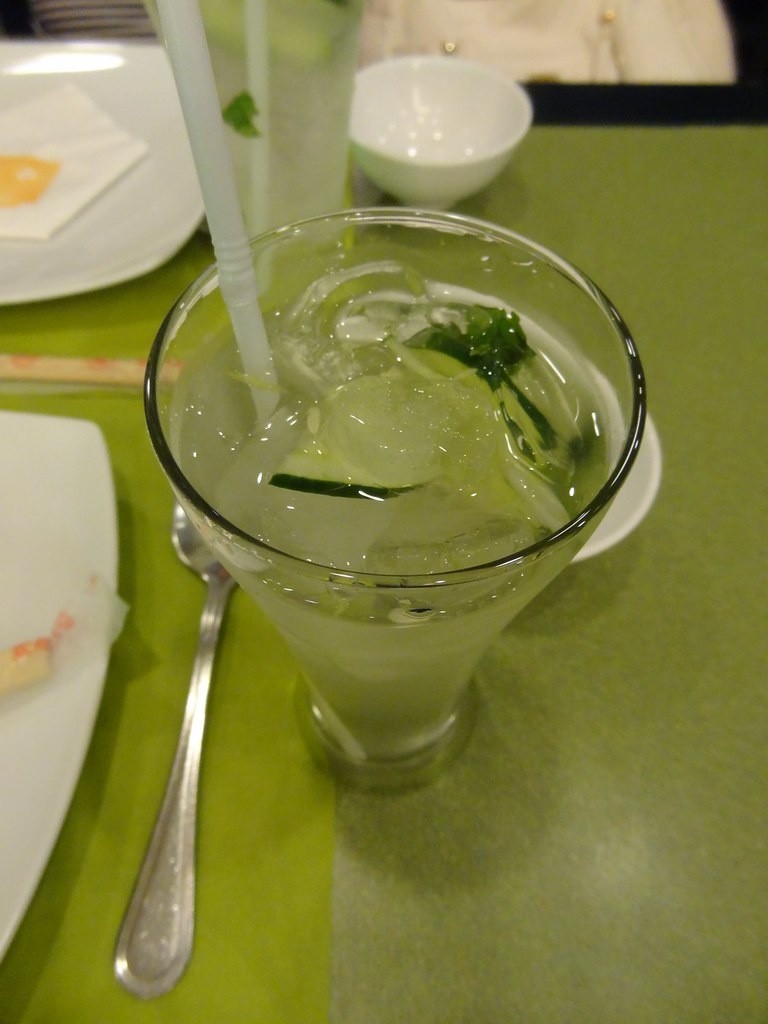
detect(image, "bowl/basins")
[349,56,533,212]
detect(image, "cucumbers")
[268,329,568,499]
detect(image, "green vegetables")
[449,304,534,364]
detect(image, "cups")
[143,207,647,791]
[143,0,364,240]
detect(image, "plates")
[430,283,660,564]
[0,410,118,965]
[1,41,205,305]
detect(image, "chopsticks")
[0,355,182,388]
